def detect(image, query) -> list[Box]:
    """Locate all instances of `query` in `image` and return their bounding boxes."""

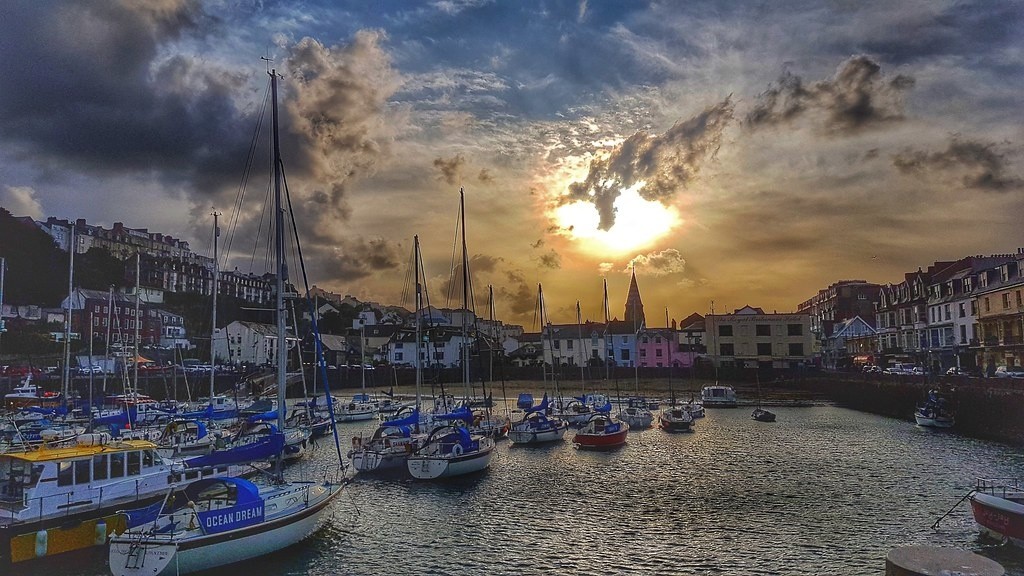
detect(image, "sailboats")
[749,369,775,422]
[655,307,696,435]
[680,343,706,418]
[0,64,663,576]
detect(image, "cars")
[861,362,928,376]
[947,367,969,376]
[994,365,1024,380]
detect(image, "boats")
[914,395,956,429]
[966,476,1024,551]
[701,382,737,405]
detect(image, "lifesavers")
[587,395,594,403]
[452,444,463,457]
[597,395,605,403]
[351,436,361,445]
